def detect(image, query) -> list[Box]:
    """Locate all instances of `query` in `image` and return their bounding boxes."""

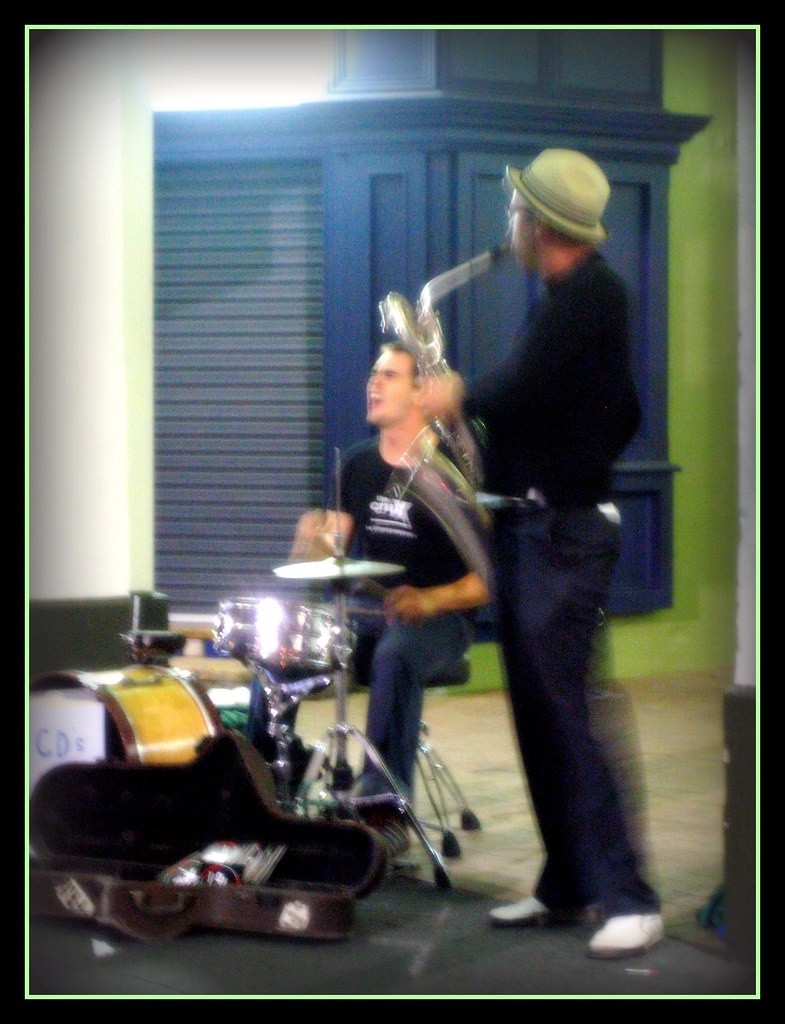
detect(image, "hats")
[506,146,608,244]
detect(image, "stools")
[356,660,480,856]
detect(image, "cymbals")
[272,554,411,583]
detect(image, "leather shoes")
[588,910,661,959]
[489,894,551,925]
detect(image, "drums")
[210,591,353,682]
[30,660,225,767]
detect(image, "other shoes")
[379,818,409,860]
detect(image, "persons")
[250,340,473,868]
[417,149,661,959]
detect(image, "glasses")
[508,201,535,221]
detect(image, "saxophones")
[377,245,523,530]
